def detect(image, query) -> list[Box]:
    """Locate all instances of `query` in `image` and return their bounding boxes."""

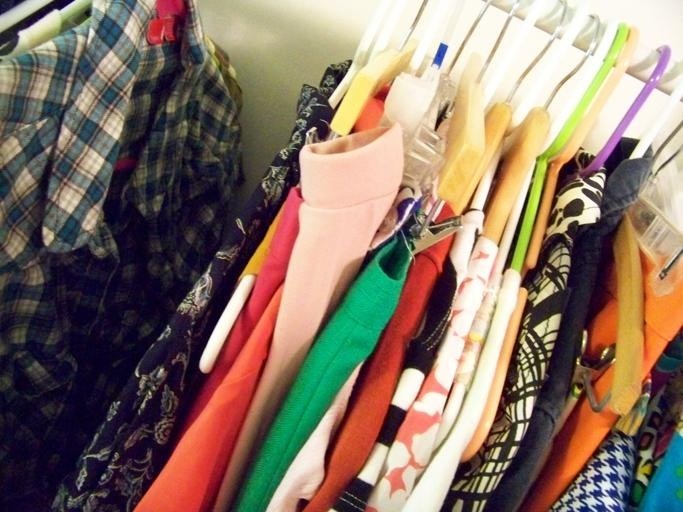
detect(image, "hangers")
[328,0,683,415]
[0,0,94,61]
